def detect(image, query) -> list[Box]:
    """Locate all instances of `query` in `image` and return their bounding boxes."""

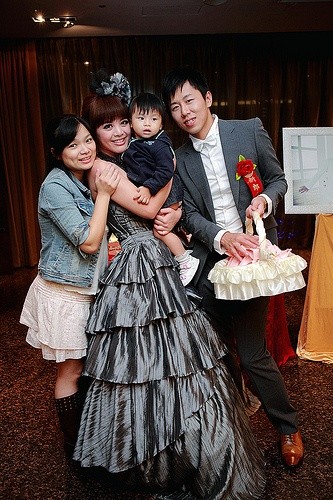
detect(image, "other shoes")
[178,256,200,287]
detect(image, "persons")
[71,72,267,500]
[19,112,121,464]
[160,66,305,470]
[121,91,200,287]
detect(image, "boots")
[54,391,99,470]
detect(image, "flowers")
[236,160,254,177]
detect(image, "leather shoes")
[280,431,305,471]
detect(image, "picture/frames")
[282,127,333,214]
